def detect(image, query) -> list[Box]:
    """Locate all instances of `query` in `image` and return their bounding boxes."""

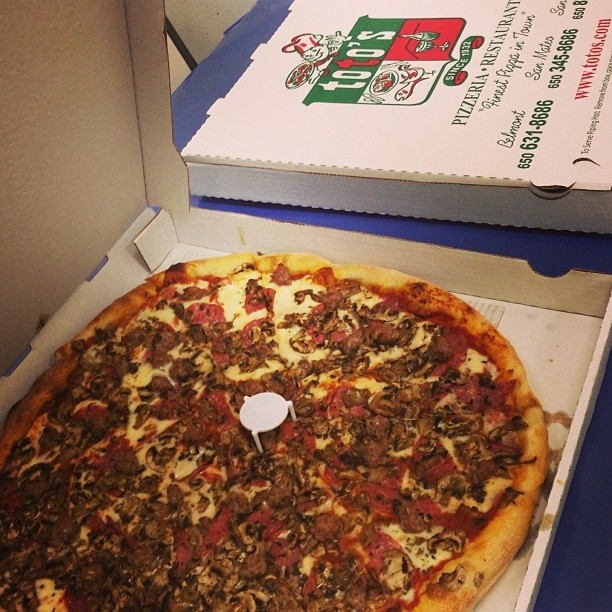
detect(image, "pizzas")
[1,253,549,611]
